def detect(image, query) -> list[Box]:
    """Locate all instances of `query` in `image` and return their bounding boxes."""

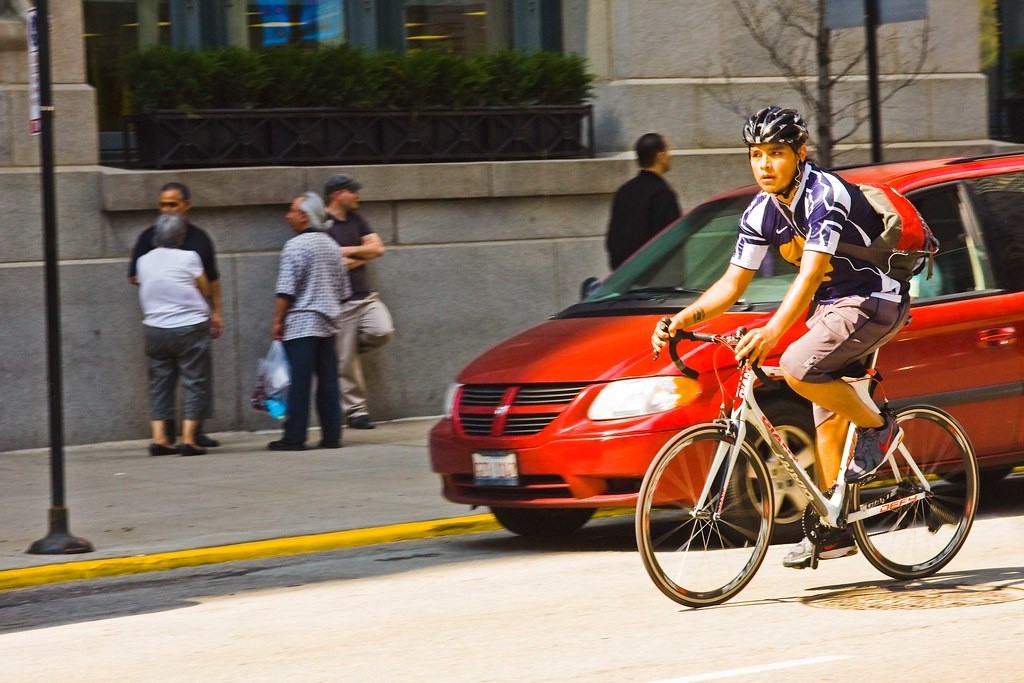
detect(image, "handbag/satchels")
[837,182,940,282]
[249,340,291,422]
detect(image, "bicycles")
[635,317,980,607]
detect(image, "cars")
[428,151,1024,535]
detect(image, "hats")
[324,174,364,193]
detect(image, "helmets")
[742,106,808,198]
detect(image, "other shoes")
[318,436,339,447]
[268,437,305,450]
[148,430,220,457]
[347,415,375,430]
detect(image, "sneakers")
[783,521,857,563]
[845,410,904,483]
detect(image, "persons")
[268,173,395,451]
[125,182,224,457]
[604,133,687,287]
[651,106,911,569]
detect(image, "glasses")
[156,200,190,208]
